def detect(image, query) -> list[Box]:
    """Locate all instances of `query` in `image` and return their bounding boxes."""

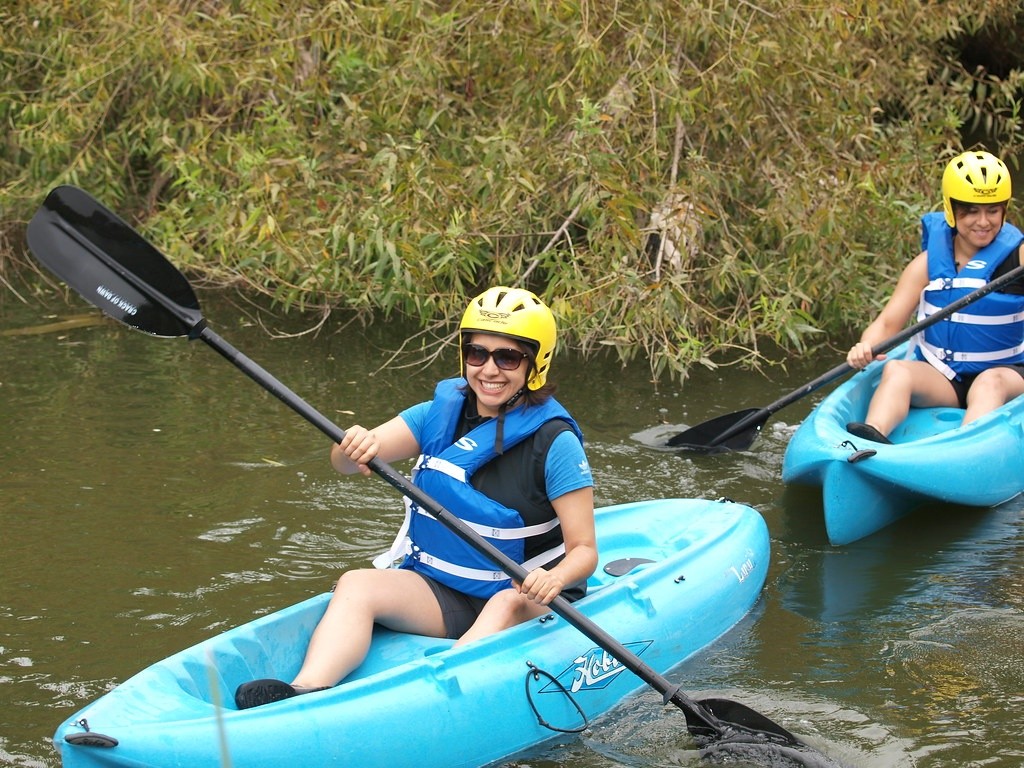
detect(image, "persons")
[847,151,1024,445]
[236,286,598,710]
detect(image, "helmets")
[942,151,1012,228]
[460,286,557,391]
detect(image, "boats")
[52,498,770,768]
[783,340,1024,547]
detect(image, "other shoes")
[846,422,893,445]
[235,680,334,710]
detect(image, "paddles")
[662,264,1024,448]
[24,187,804,748]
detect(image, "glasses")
[462,343,528,370]
